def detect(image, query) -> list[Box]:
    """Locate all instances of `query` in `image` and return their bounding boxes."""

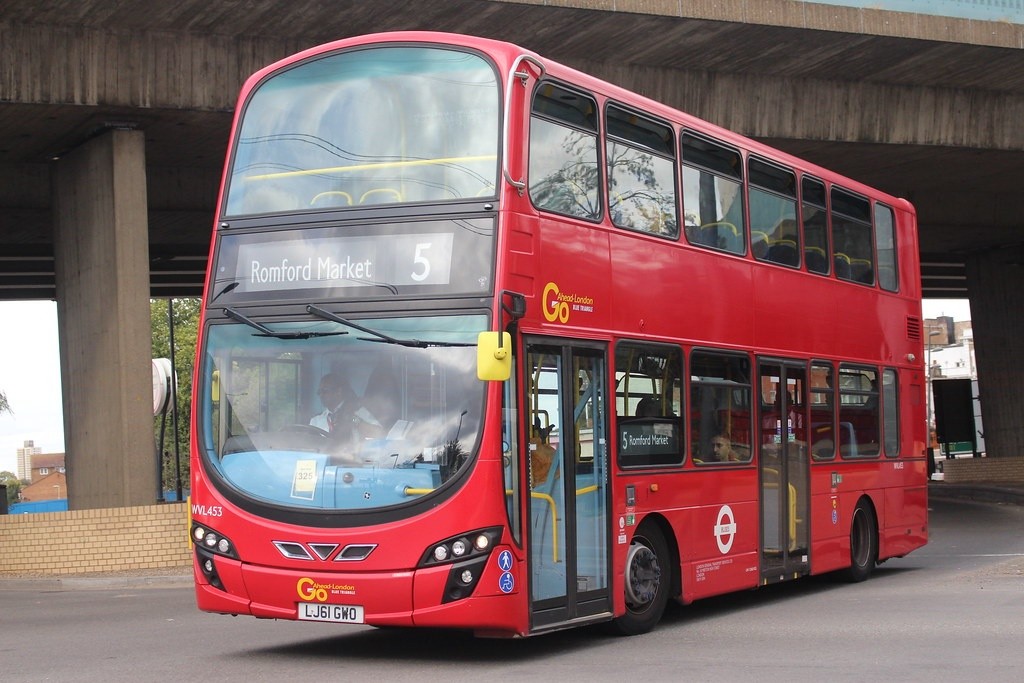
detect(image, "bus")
[185,28,931,640]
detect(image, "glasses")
[317,386,330,394]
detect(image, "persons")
[309,372,387,454]
[586,389,802,462]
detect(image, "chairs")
[731,441,879,461]
[311,189,894,297]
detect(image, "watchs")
[351,416,361,426]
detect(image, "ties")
[327,413,334,432]
